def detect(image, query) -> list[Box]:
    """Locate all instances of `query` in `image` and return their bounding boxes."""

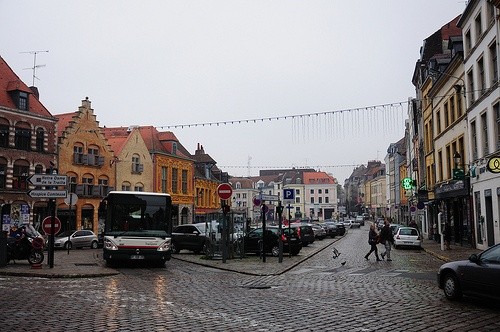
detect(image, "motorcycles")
[1,226,44,265]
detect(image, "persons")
[409,220,419,235]
[363,221,393,261]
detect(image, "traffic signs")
[25,190,68,199]
[26,175,67,186]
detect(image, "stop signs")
[217,184,232,199]
[42,216,61,235]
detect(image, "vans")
[195,221,246,243]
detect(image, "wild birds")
[332,247,342,259]
[341,261,346,266]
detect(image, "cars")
[437,243,500,300]
[44,230,99,250]
[376,217,420,249]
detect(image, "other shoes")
[376,258,382,261]
[364,256,370,260]
[387,258,392,261]
[380,253,385,259]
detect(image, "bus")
[99,191,178,265]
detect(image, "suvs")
[172,225,216,254]
[339,211,369,228]
[233,218,345,257]
